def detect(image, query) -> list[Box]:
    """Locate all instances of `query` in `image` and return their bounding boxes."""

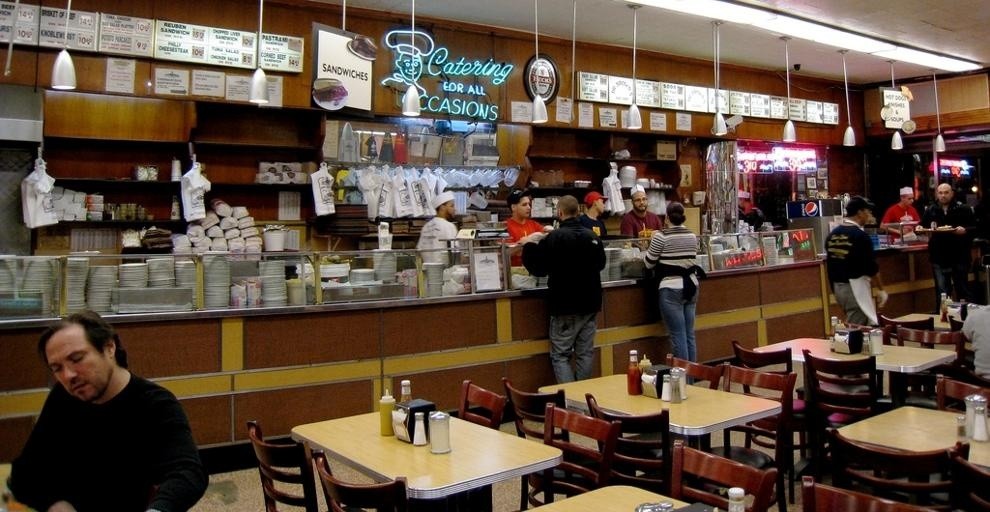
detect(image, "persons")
[577,191,608,237]
[497,190,555,267]
[415,190,458,265]
[517,194,606,384]
[825,195,883,328]
[643,201,700,385]
[912,182,977,316]
[879,186,921,244]
[962,305,990,382]
[738,191,766,233]
[5,307,209,512]
[619,184,662,236]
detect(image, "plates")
[24,257,57,313]
[87,265,118,311]
[422,263,443,297]
[762,236,778,266]
[65,258,90,312]
[146,257,176,287]
[351,269,375,283]
[710,244,723,254]
[373,253,396,281]
[258,260,287,307]
[0,255,16,297]
[203,255,229,310]
[119,263,147,288]
[600,247,622,282]
[696,255,710,271]
[176,261,199,310]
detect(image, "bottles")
[662,375,671,401]
[640,355,652,370]
[670,369,682,403]
[869,329,884,355]
[940,293,948,322]
[628,350,642,395]
[672,367,688,399]
[429,412,451,454]
[401,380,413,402]
[829,316,838,352]
[171,156,182,181]
[973,407,988,442]
[862,332,870,356]
[379,390,396,436]
[414,413,427,446]
[728,487,745,511]
[171,195,180,220]
[956,417,967,439]
[964,394,988,438]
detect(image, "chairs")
[700,362,798,512]
[583,393,669,497]
[501,379,600,509]
[732,341,806,466]
[458,378,505,511]
[828,428,970,506]
[879,314,935,348]
[896,326,963,403]
[961,364,990,388]
[247,421,318,512]
[797,348,880,475]
[540,402,622,503]
[311,449,407,512]
[800,476,935,511]
[664,356,724,474]
[944,443,990,508]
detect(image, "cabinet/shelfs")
[500,119,704,241]
[180,99,324,268]
[306,116,511,255]
[28,91,193,263]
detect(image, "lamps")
[886,60,903,149]
[248,1,268,103]
[781,37,796,142]
[838,49,856,147]
[50,1,78,89]
[403,0,421,117]
[625,4,643,130]
[929,68,946,152]
[531,1,549,123]
[711,22,728,135]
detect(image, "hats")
[432,190,454,208]
[847,195,875,216]
[630,183,646,194]
[506,190,529,205]
[899,186,914,195]
[583,191,610,205]
